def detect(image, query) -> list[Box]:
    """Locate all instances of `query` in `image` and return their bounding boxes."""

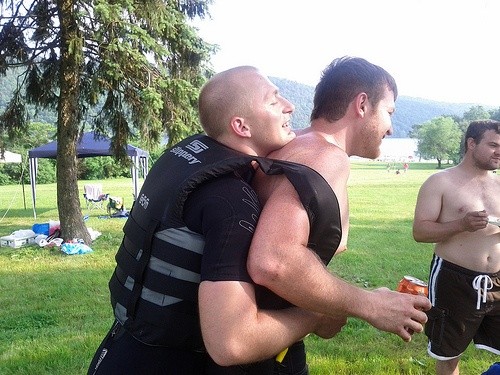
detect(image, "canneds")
[398,274,428,335]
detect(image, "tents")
[28,129,150,220]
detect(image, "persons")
[386,161,410,175]
[412,119,500,375]
[85,62,350,374]
[246,53,432,374]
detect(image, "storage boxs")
[1,234,38,249]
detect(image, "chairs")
[83,184,110,210]
